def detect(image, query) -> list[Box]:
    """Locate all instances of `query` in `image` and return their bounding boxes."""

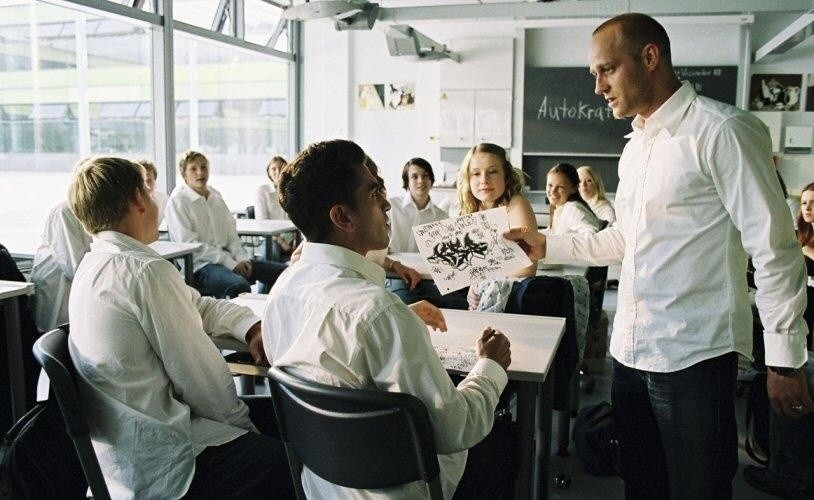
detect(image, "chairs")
[267,366,447,499]
[32,327,115,500]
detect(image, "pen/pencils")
[488,330,495,339]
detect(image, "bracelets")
[390,259,401,273]
[279,238,285,245]
[767,361,809,376]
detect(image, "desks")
[236,218,299,261]
[0,279,35,425]
[390,253,587,285]
[207,292,566,500]
[147,240,204,287]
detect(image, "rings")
[790,402,805,412]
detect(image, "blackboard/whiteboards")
[522,65,738,195]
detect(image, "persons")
[66,156,297,500]
[31,199,92,338]
[254,157,302,264]
[386,157,452,311]
[794,182,814,277]
[502,11,813,499]
[166,151,290,299]
[366,157,420,290]
[747,155,790,292]
[259,138,511,500]
[131,158,169,226]
[537,161,600,268]
[454,142,538,277]
[576,166,615,226]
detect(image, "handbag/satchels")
[0,403,88,499]
[572,401,619,475]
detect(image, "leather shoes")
[743,465,789,496]
[745,428,770,465]
